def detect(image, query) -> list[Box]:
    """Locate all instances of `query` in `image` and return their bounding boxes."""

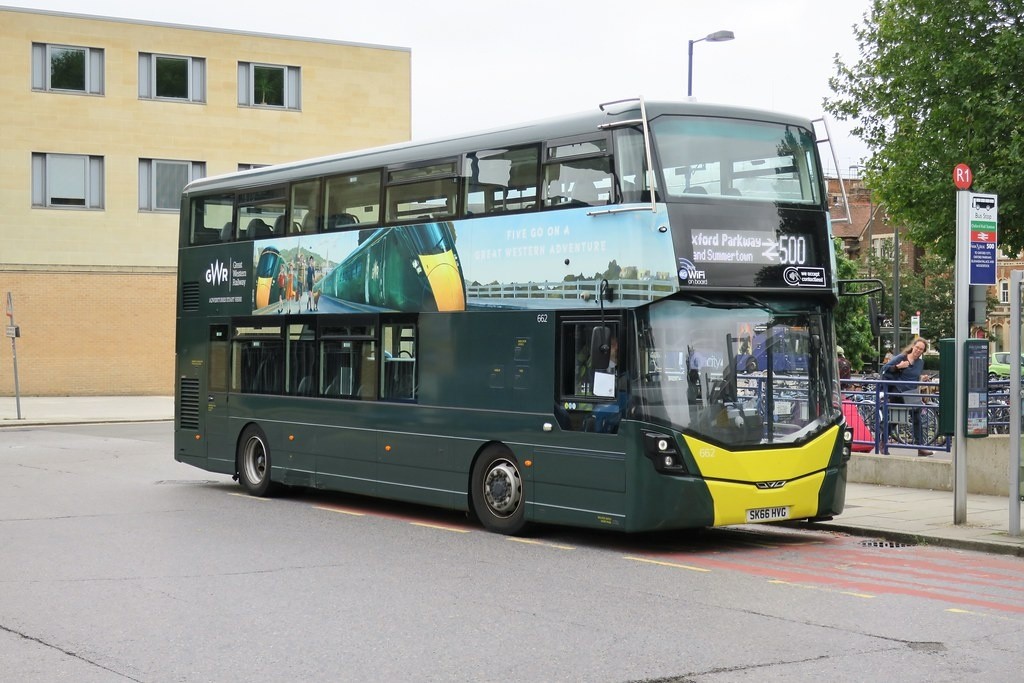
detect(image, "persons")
[277,256,316,314]
[584,336,627,429]
[879,338,934,456]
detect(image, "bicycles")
[848,374,1011,449]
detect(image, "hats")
[836,345,844,354]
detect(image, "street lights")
[685,31,741,102]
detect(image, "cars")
[988,351,1024,376]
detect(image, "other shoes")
[278,302,314,314]
[917,450,934,456]
[879,441,890,455]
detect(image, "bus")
[175,96,888,537]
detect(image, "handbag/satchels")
[880,353,906,391]
[903,388,924,410]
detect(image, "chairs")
[241,340,418,400]
[569,176,743,205]
[221,211,360,240]
